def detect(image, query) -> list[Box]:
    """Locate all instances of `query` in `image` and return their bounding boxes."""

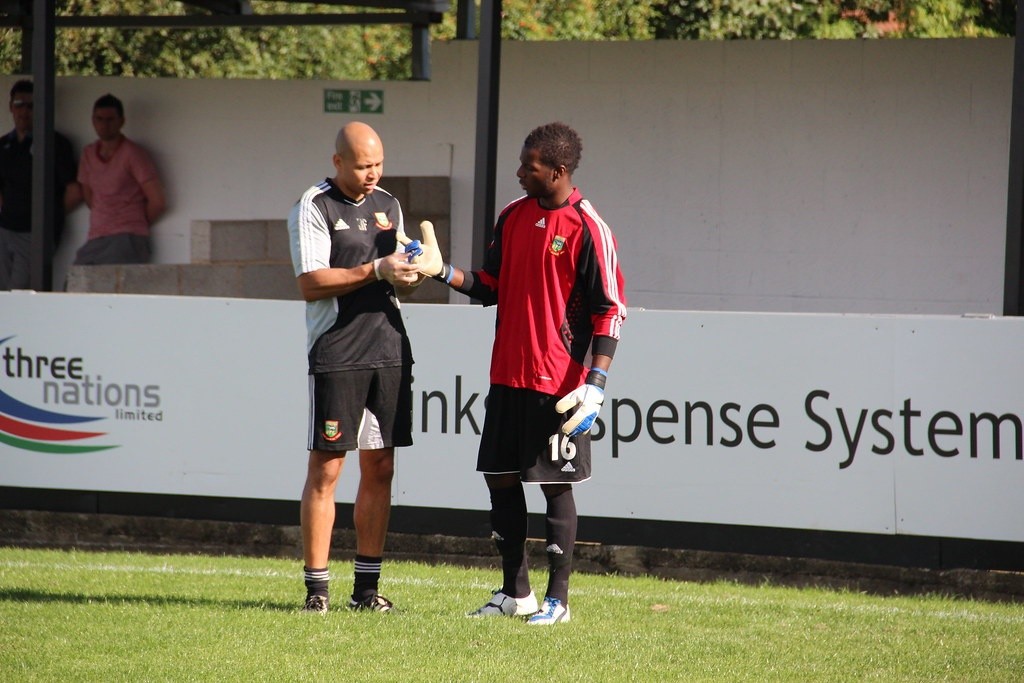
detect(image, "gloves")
[396,220,454,284]
[555,368,608,439]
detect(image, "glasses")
[13,99,33,108]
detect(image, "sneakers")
[350,594,393,613]
[465,586,539,616]
[300,595,328,614]
[524,597,570,626]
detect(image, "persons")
[289,118,426,614]
[0,77,80,291]
[392,122,626,628]
[76,92,169,264]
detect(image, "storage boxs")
[66,174,449,306]
